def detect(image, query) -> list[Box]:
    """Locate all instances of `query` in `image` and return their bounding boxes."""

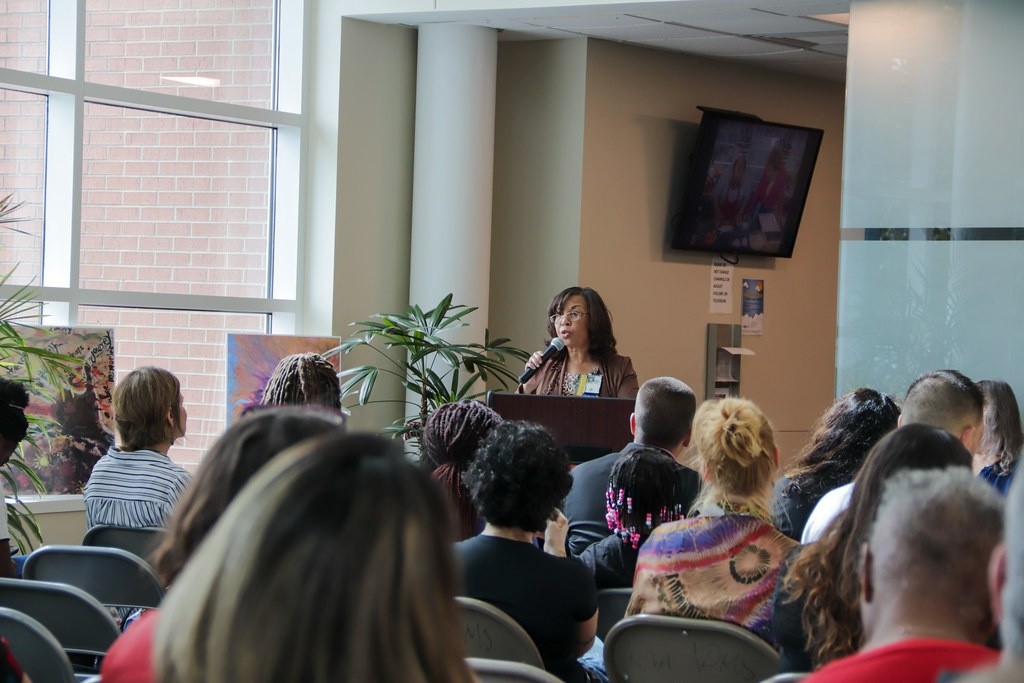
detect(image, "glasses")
[549,311,588,324]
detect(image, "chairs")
[0,524,167,683]
[455,587,814,683]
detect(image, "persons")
[0,353,600,683]
[705,133,793,226]
[563,369,1024,683]
[514,287,640,399]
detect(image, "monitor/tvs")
[670,111,825,258]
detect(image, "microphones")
[518,338,565,385]
